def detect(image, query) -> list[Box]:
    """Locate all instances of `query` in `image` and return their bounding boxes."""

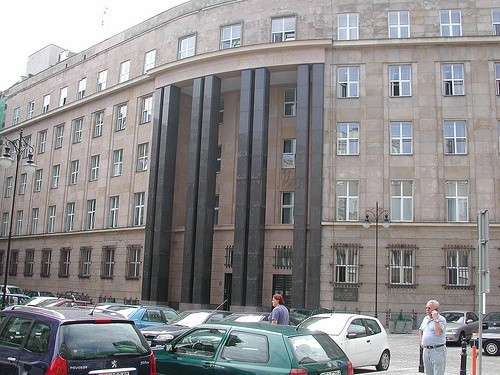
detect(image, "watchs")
[434,320,439,323]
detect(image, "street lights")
[362,201,390,319]
[0,127,37,308]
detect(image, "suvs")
[0,304,157,375]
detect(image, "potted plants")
[388,310,413,334]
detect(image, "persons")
[419,300,447,375]
[268,294,289,325]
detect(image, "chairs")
[9,321,40,351]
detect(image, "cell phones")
[434,309,437,311]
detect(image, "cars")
[0,285,30,310]
[297,313,391,372]
[0,295,123,335]
[140,309,234,348]
[482,311,500,330]
[150,322,353,375]
[288,307,332,319]
[216,312,301,328]
[439,310,479,346]
[469,327,500,356]
[106,305,179,329]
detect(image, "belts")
[424,344,444,349]
